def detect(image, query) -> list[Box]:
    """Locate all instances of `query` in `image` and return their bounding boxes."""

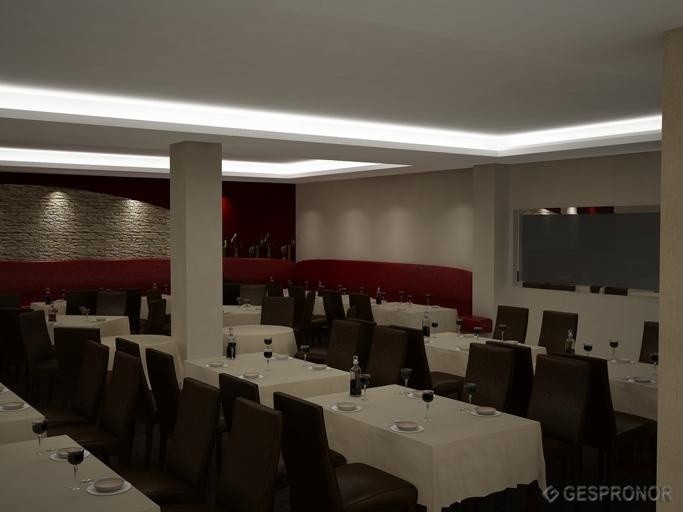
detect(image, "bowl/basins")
[616,358,631,363]
[459,346,470,351]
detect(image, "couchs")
[283,260,493,334]
[0,257,295,311]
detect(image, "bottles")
[375,288,382,305]
[349,356,362,397]
[565,329,575,354]
[225,328,237,359]
[422,311,431,338]
[44,287,51,305]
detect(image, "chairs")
[388,325,465,400]
[345,317,377,374]
[260,296,294,328]
[365,326,413,388]
[54,328,100,360]
[551,352,657,486]
[493,305,529,343]
[348,292,375,320]
[538,310,578,354]
[1,309,33,385]
[120,378,221,506]
[48,351,140,472]
[95,290,127,316]
[525,353,590,512]
[325,319,362,372]
[19,310,57,407]
[147,288,162,306]
[121,287,141,336]
[66,288,97,315]
[486,341,534,418]
[223,285,241,306]
[273,391,418,512]
[267,282,283,296]
[287,285,327,345]
[145,348,226,471]
[116,337,157,473]
[0,293,19,308]
[639,322,659,363]
[161,397,283,512]
[219,373,347,489]
[143,298,167,334]
[39,339,109,427]
[460,343,518,413]
[322,289,347,331]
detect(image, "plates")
[308,366,330,371]
[94,477,124,492]
[390,425,424,433]
[0,389,9,394]
[456,347,469,354]
[312,364,326,370]
[336,401,357,410]
[4,402,25,410]
[477,407,496,414]
[208,361,224,367]
[0,404,30,412]
[471,410,501,416]
[397,422,417,431]
[243,372,259,378]
[86,481,132,496]
[57,447,72,458]
[239,375,264,381]
[49,450,90,462]
[610,360,635,364]
[205,364,228,368]
[329,403,362,413]
[636,376,650,383]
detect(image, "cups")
[465,383,476,409]
[263,348,272,371]
[584,343,594,357]
[80,307,85,319]
[401,368,412,395]
[609,338,618,359]
[264,335,272,350]
[421,390,434,421]
[474,327,483,342]
[432,320,439,338]
[66,447,84,491]
[83,309,90,319]
[407,295,413,306]
[456,319,463,336]
[381,292,386,304]
[360,374,371,401]
[650,352,657,370]
[399,290,404,304]
[499,324,505,342]
[301,345,308,367]
[32,418,48,455]
[425,293,431,307]
